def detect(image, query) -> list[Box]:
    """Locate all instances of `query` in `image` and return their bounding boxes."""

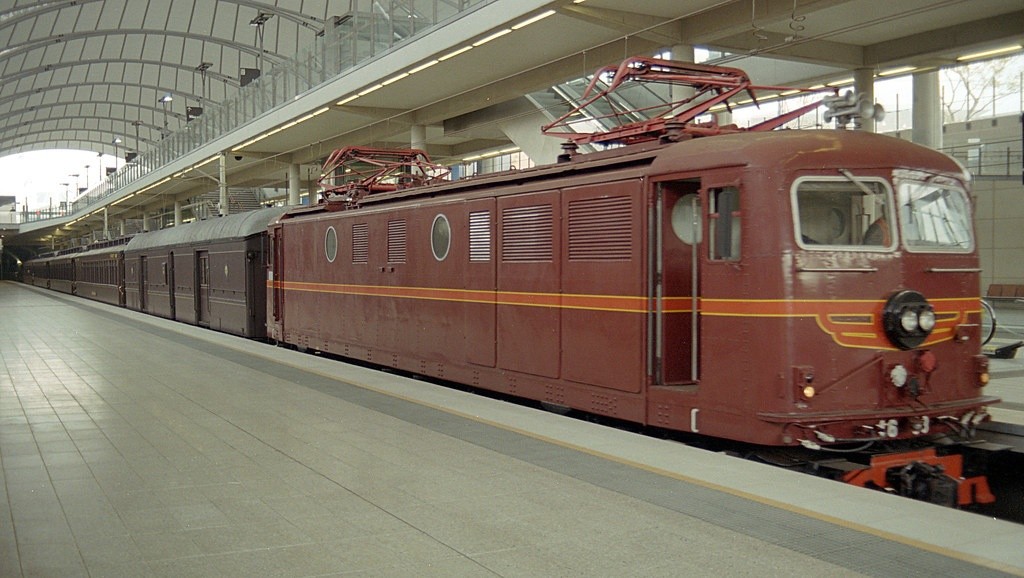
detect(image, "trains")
[21,54,1023,518]
